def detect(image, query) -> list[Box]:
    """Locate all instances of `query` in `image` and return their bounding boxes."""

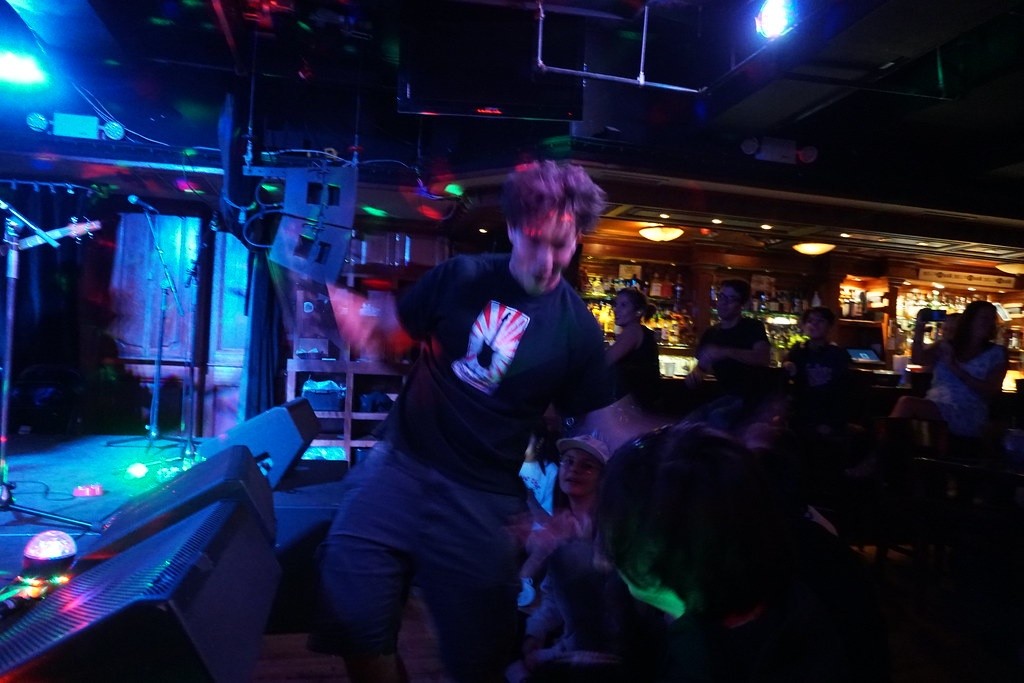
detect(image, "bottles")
[585,274,695,345]
[896,293,993,355]
[752,290,820,314]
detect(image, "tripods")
[99,209,204,449]
[0,201,106,535]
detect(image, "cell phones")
[927,309,946,321]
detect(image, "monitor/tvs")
[845,347,881,361]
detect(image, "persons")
[297,160,888,683]
[890,301,1005,435]
[15,297,145,435]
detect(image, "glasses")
[716,293,742,303]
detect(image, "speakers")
[0,396,323,683]
[269,166,358,286]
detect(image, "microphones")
[128,195,156,211]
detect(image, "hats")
[557,435,610,464]
